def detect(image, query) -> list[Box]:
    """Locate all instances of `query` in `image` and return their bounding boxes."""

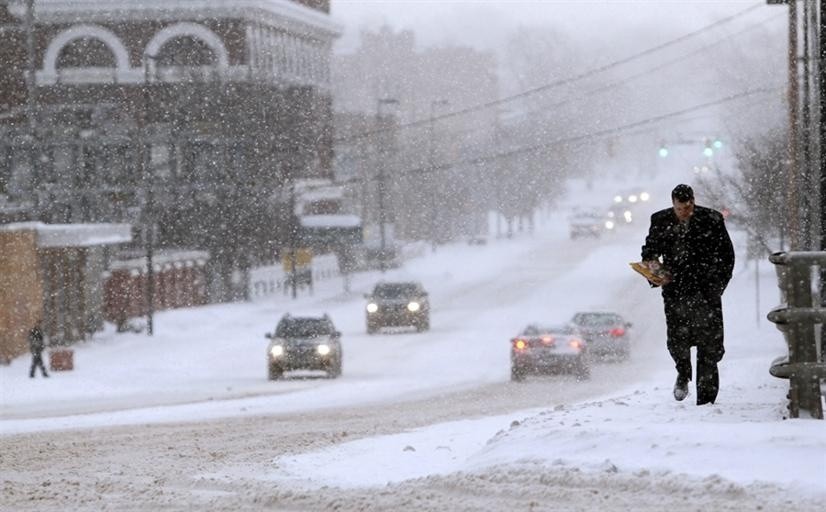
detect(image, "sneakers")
[674,374,689,401]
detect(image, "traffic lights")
[704,148,712,158]
[714,141,721,149]
[659,148,668,158]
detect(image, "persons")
[27,320,50,379]
[641,184,735,406]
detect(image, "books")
[629,259,669,287]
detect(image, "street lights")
[430,98,448,141]
[143,49,184,336]
[375,95,398,252]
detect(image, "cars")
[365,282,429,332]
[509,315,591,382]
[569,209,603,238]
[265,314,342,379]
[573,313,634,362]
